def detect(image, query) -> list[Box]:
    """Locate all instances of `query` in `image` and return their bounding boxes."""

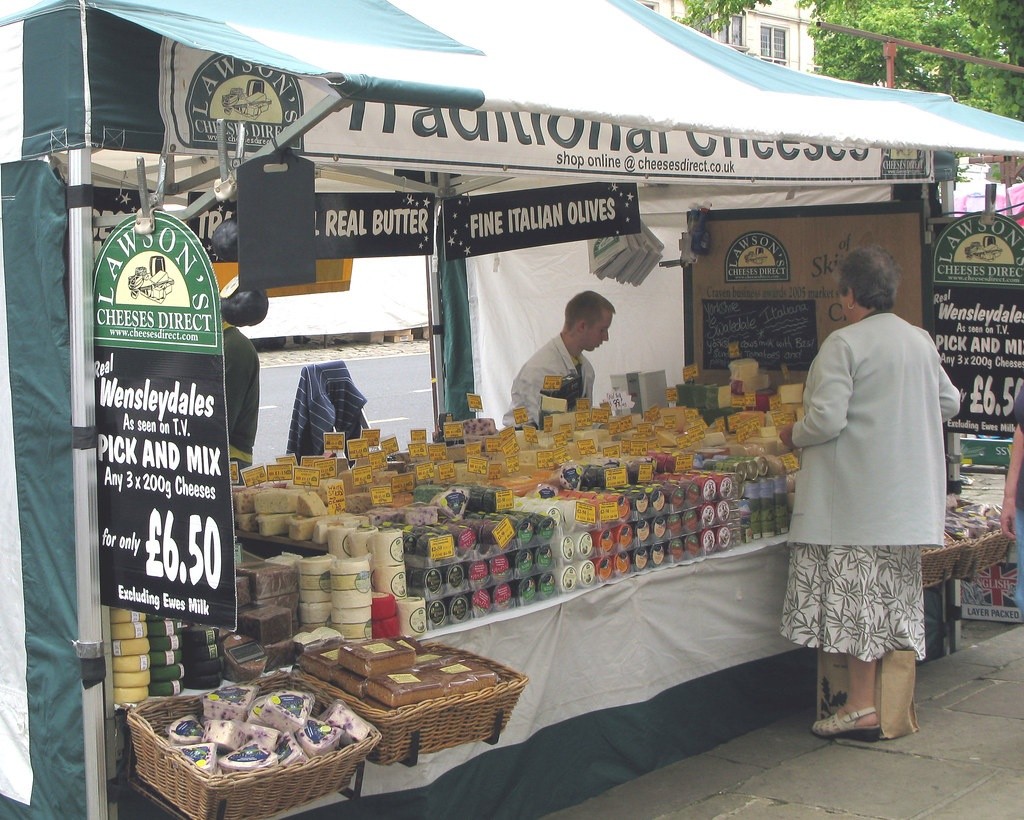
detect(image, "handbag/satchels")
[816,647,920,740]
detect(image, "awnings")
[84,0,1023,223]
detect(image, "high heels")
[812,707,880,743]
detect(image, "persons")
[1000,385,1024,621]
[780,247,960,739]
[503,292,614,429]
[223,316,260,485]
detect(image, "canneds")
[393,454,790,628]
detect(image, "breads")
[109,453,428,703]
[167,684,371,779]
[428,357,806,496]
[300,635,500,713]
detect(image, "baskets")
[125,668,382,820]
[291,642,528,768]
[954,528,1012,578]
[921,541,961,588]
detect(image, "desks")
[118,532,943,820]
